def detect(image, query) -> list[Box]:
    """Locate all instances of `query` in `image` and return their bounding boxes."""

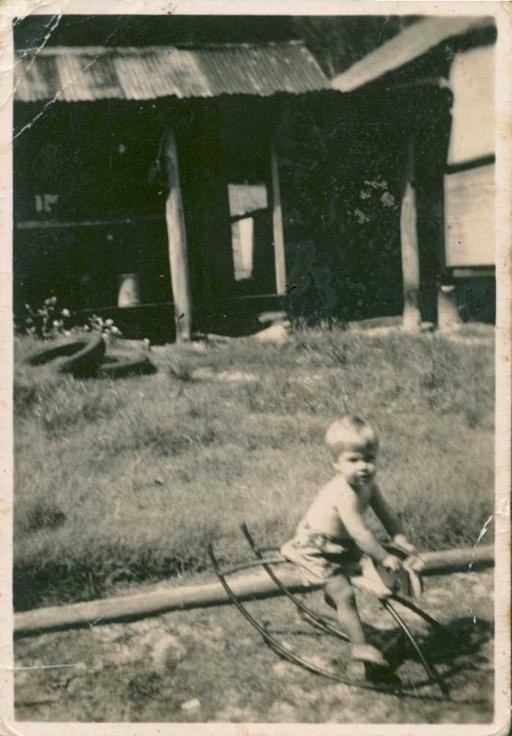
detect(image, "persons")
[282,416,417,667]
